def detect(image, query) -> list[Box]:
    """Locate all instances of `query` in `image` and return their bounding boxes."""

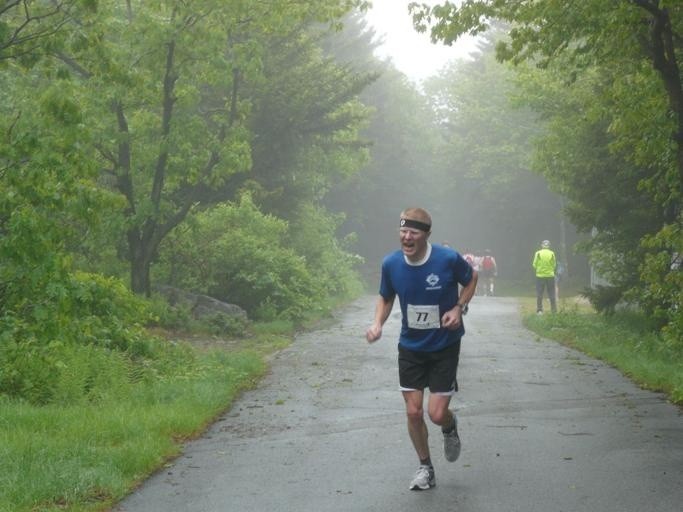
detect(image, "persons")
[362,205,479,491]
[441,242,448,248]
[480,247,497,297]
[458,246,474,269]
[546,249,564,304]
[531,239,556,315]
[472,249,481,296]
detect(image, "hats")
[541,239,550,248]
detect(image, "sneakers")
[442,415,460,462]
[409,464,436,490]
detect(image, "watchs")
[455,303,468,315]
